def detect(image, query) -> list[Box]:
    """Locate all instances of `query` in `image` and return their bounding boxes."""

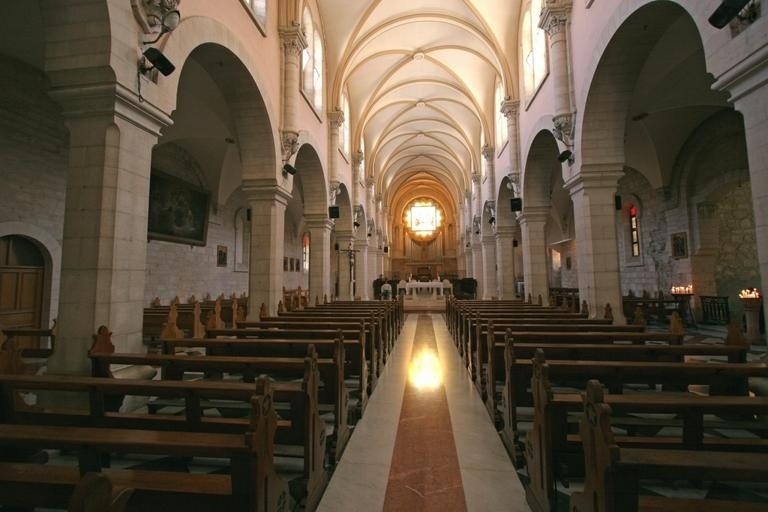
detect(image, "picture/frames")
[671,231,688,259]
[148,166,212,246]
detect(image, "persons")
[372,274,397,300]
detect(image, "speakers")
[329,207,341,219]
[248,209,251,220]
[614,195,622,210]
[510,199,522,213]
[335,244,339,251]
[513,239,518,248]
[384,247,388,253]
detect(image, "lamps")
[553,129,574,147]
[142,9,180,44]
[282,143,298,161]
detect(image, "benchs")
[569,379,765,511]
[84,287,400,461]
[526,348,766,510]
[445,290,700,424]
[499,327,766,444]
[2,373,292,510]
[2,342,331,510]
